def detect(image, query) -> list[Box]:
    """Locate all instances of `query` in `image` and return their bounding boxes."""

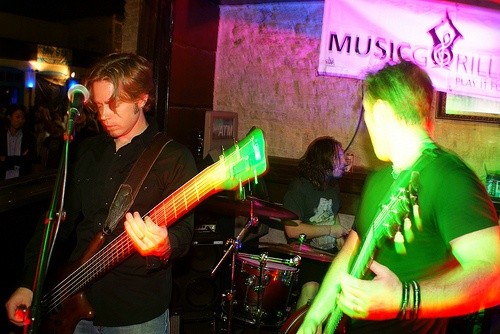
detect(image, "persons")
[283,137,357,281]
[0,100,99,178]
[5,52,198,334]
[297,58,500,334]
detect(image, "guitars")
[19,125,269,334]
[308,172,419,334]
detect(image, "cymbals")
[257,239,336,264]
[198,190,300,221]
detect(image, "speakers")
[169,238,233,334]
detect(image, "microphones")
[67,84,90,132]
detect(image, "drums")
[219,245,299,328]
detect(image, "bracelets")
[397,280,422,322]
